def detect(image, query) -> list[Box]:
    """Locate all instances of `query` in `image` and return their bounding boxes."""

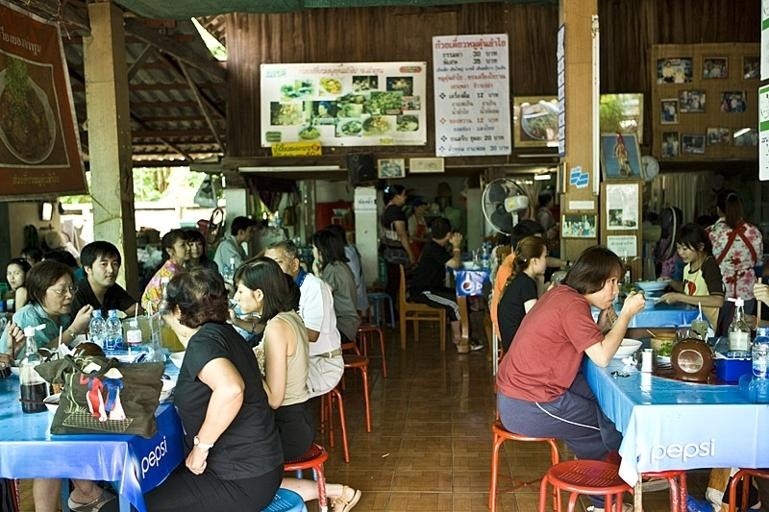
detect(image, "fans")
[197,207,224,257]
[480,178,534,251]
[642,205,678,282]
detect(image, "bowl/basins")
[167,352,185,369]
[461,261,476,268]
[9,359,46,378]
[613,338,642,360]
[649,333,678,355]
[42,393,61,415]
[157,379,176,407]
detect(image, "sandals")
[331,485,361,512]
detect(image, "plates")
[271,76,419,141]
[642,297,661,310]
[521,105,560,141]
[636,280,672,292]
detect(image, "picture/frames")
[601,92,644,144]
[600,132,645,182]
[513,96,558,148]
[660,55,759,158]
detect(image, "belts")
[321,350,341,358]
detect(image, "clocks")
[640,154,660,182]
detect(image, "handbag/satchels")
[384,248,409,264]
[34,354,165,439]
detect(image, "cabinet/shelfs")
[424,212,444,232]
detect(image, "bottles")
[690,319,708,343]
[725,296,769,397]
[87,309,105,347]
[640,375,652,401]
[221,257,236,282]
[19,323,50,412]
[103,308,123,356]
[481,245,490,271]
[125,317,143,364]
[641,348,656,374]
[155,275,169,311]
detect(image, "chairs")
[715,296,744,337]
[399,264,447,353]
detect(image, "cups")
[471,249,482,269]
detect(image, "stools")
[492,322,502,377]
[263,290,396,511]
[729,468,769,512]
[488,382,561,512]
[608,450,688,512]
[547,460,632,512]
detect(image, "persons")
[1,203,372,512]
[379,183,573,355]
[703,187,765,303]
[663,206,684,283]
[753,281,769,309]
[662,223,726,330]
[493,244,646,511]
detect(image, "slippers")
[68,491,116,512]
